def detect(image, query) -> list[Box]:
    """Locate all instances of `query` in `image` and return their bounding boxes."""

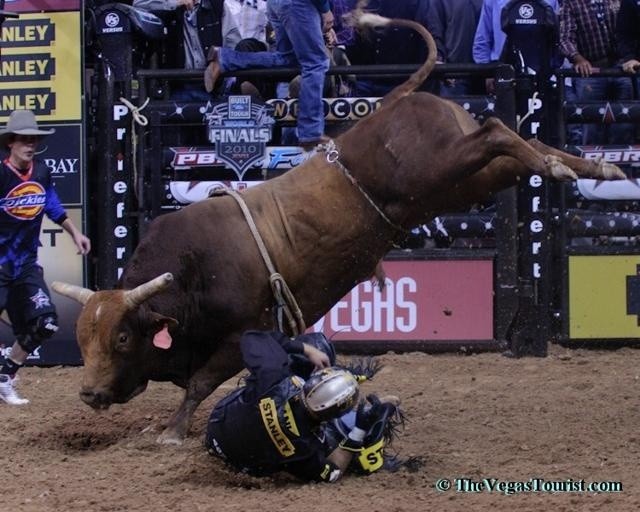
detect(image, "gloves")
[340,394,379,453]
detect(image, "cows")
[50,7,628,447]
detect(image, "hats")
[1,109,56,136]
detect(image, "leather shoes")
[202,46,225,93]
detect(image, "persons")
[0,108,92,407]
[204,320,404,487]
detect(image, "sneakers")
[1,345,21,406]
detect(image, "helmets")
[300,366,359,422]
